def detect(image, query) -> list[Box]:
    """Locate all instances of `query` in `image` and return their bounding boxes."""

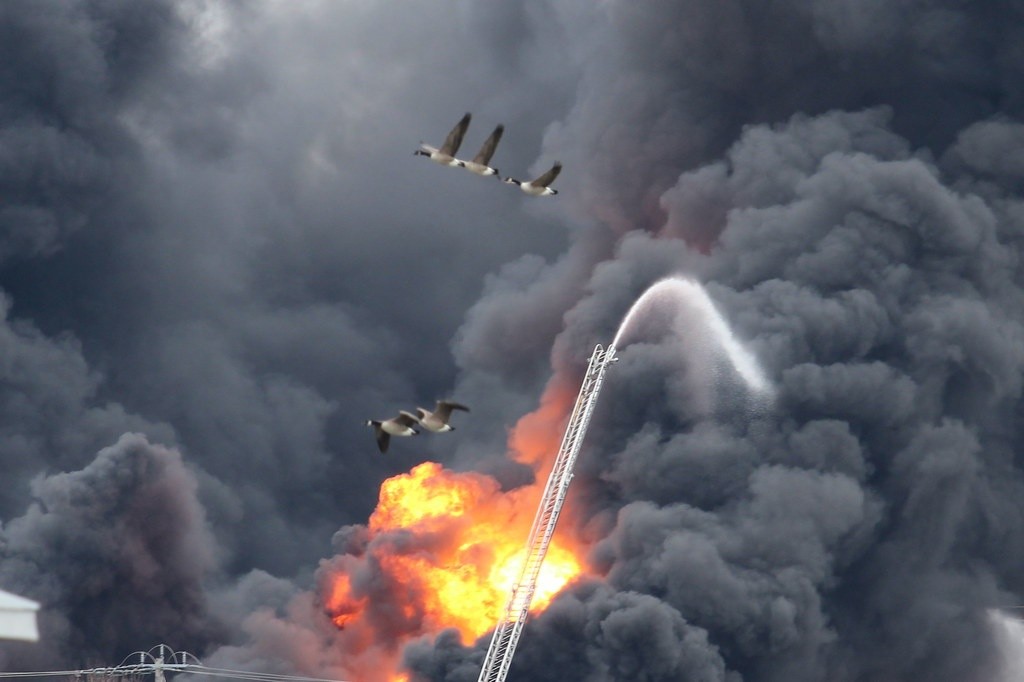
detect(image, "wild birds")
[363,398,471,455]
[412,110,506,182]
[503,160,564,197]
